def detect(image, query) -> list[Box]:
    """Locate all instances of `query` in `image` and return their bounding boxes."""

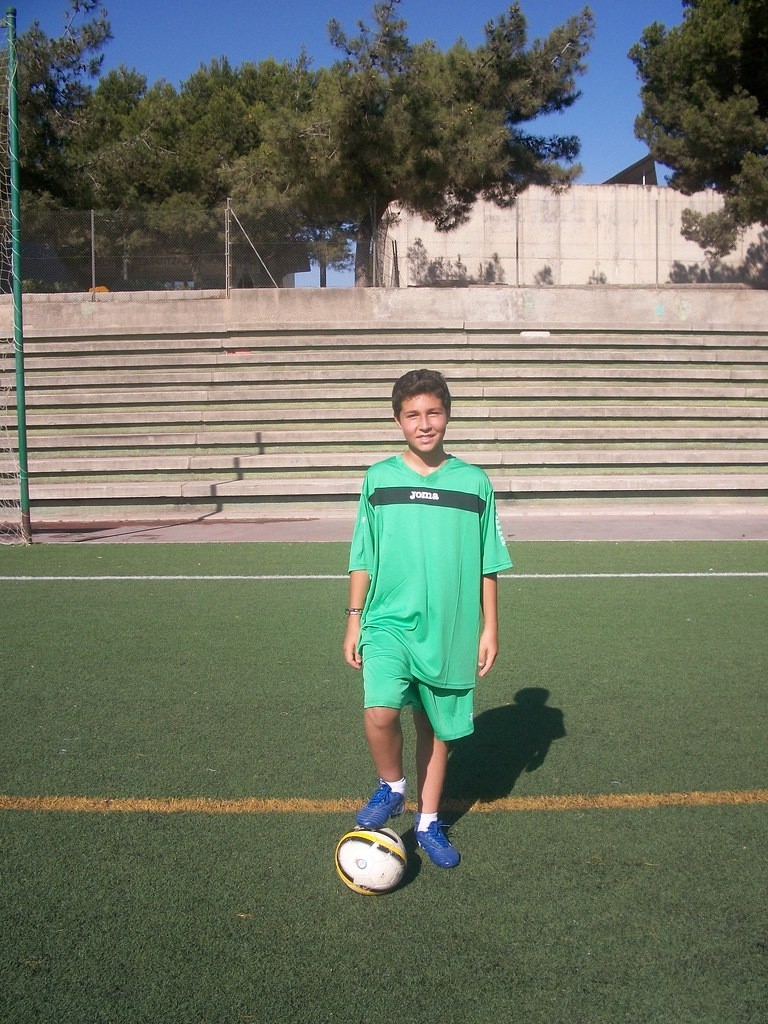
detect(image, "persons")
[343,369,513,868]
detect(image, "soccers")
[335,824,408,896]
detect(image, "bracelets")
[344,608,363,616]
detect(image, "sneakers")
[356,780,404,828]
[412,821,460,868]
